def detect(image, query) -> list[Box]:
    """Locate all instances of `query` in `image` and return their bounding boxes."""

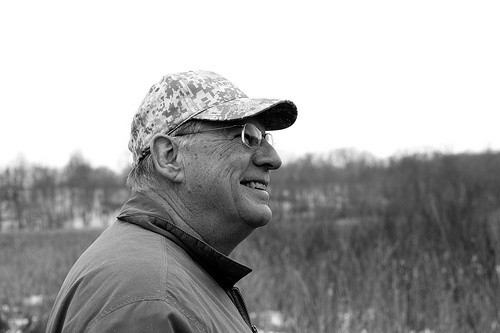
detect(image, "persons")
[45,71,297,333]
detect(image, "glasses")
[138,122,274,161]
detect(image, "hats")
[129,70,297,165]
